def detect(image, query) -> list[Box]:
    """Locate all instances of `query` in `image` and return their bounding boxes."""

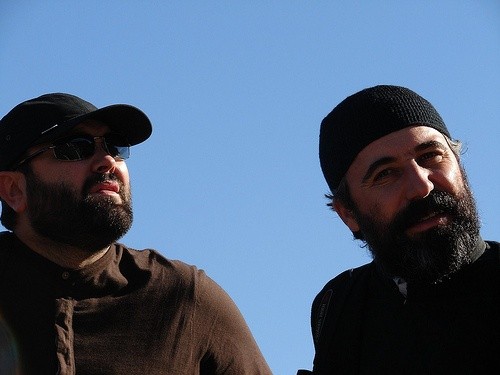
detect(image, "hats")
[319,85,453,193]
[0,93,153,171]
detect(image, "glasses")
[15,134,130,170]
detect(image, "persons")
[297,85,500,375]
[0,93,274,375]
